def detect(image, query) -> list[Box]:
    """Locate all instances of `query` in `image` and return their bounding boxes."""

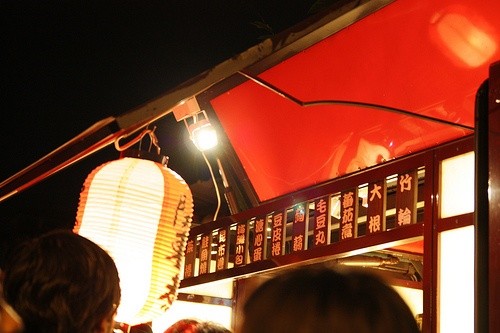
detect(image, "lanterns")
[73,155,194,324]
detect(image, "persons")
[240,261,420,332]
[2,230,121,332]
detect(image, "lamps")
[71,127,197,333]
